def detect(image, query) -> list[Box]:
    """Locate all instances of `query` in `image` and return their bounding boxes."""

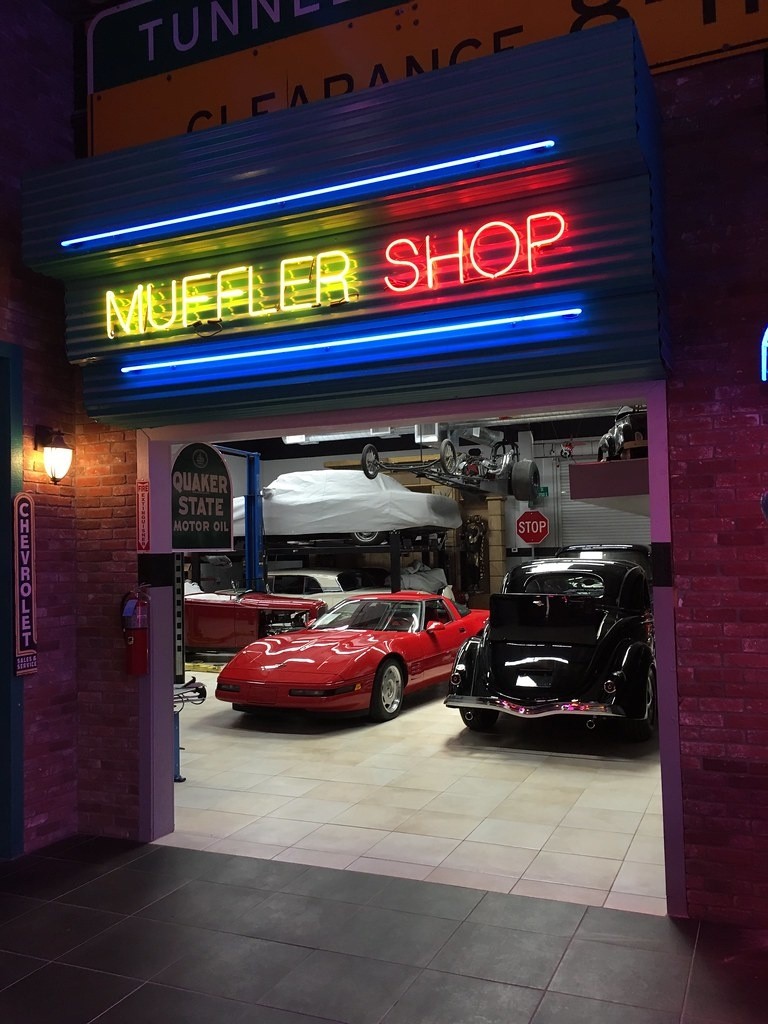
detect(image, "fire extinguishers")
[121,582,152,677]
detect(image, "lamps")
[34,424,79,485]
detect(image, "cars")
[234,470,464,547]
[597,404,648,462]
[184,580,329,652]
[215,591,489,722]
[444,559,656,743]
[554,543,652,579]
[266,569,390,611]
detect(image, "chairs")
[422,606,446,628]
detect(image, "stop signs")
[516,511,548,545]
